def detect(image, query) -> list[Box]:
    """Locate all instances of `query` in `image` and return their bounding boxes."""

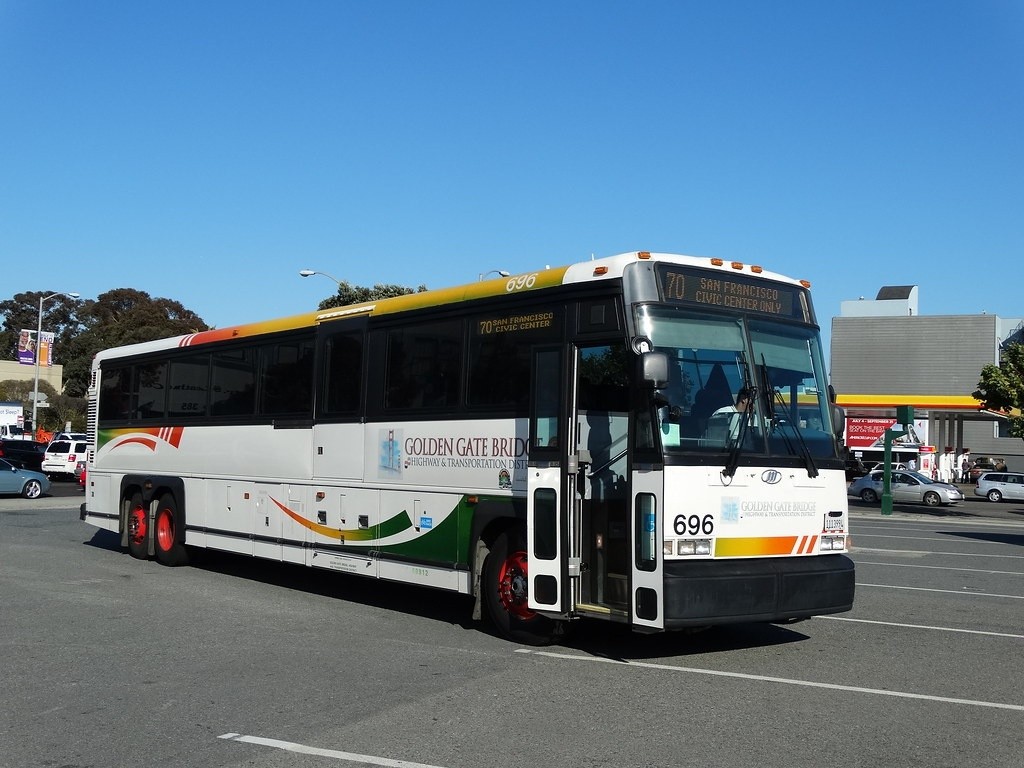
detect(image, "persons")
[900,459,917,471]
[19,331,37,365]
[962,459,970,484]
[713,387,776,449]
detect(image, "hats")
[737,387,751,403]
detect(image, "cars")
[973,472,1024,502]
[0,456,50,500]
[862,461,883,472]
[0,438,51,473]
[868,463,907,480]
[969,463,997,483]
[847,469,966,507]
[846,459,868,479]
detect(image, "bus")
[92,250,858,647]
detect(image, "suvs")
[52,432,88,441]
[40,439,88,483]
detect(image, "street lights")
[31,291,81,441]
